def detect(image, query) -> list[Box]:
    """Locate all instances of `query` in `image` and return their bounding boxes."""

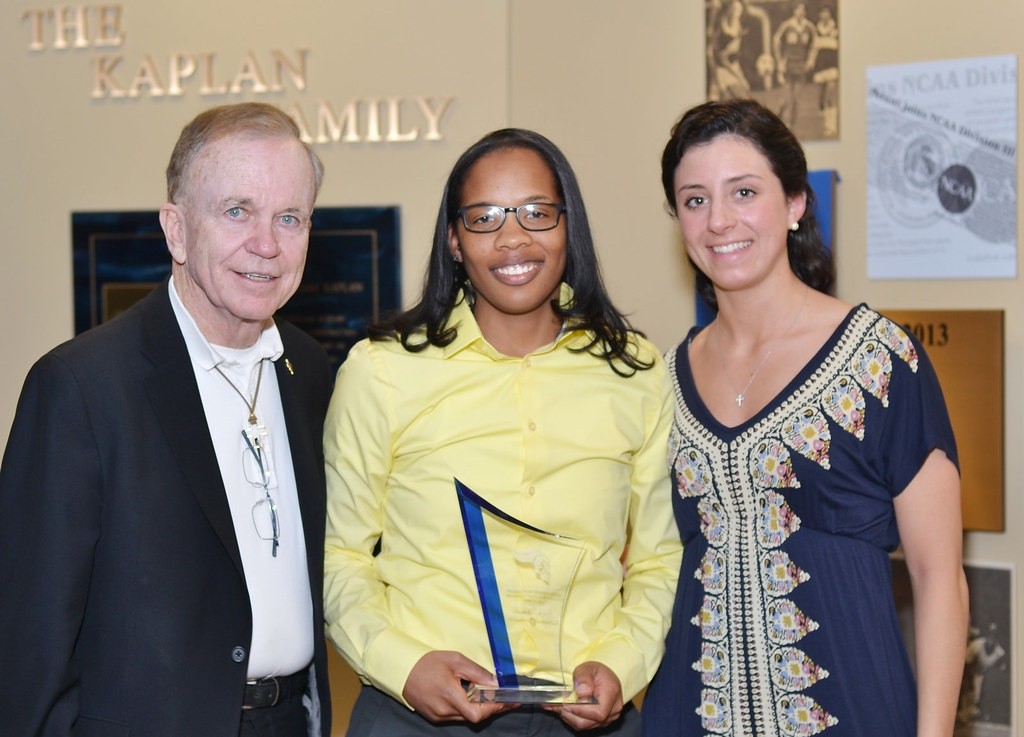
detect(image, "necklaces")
[214,358,268,449]
[716,285,810,407]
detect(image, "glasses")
[455,200,569,232]
[242,428,281,557]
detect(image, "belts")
[241,667,310,710]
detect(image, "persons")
[956,622,1007,726]
[2,104,333,737]
[322,128,684,737]
[704,1,838,138]
[663,99,969,737]
